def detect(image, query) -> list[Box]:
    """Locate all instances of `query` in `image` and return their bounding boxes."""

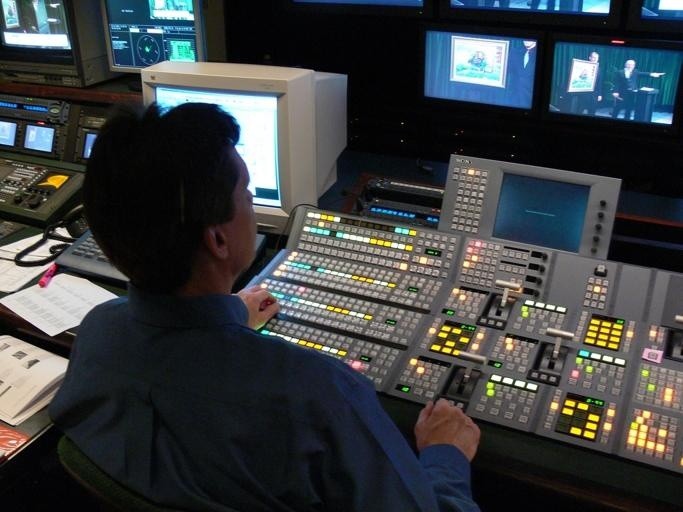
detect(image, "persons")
[574,51,603,117]
[608,59,667,121]
[42,101,483,511]
[507,40,536,109]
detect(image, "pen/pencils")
[38,263,59,287]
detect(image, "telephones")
[63,203,91,238]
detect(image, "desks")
[0,172,683,511]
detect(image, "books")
[0,334,71,428]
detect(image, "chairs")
[57,435,178,511]
